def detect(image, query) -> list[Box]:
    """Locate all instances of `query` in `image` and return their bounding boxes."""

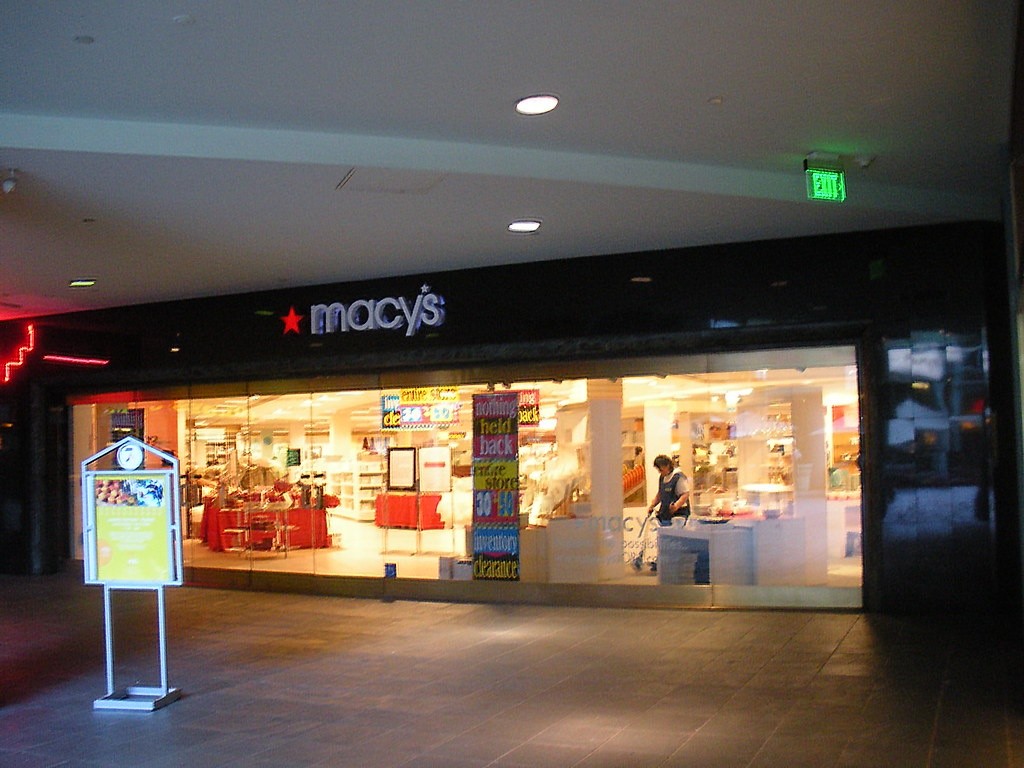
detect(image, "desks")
[464,519,598,585]
[656,515,804,586]
[375,490,445,531]
[197,492,339,553]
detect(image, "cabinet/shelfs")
[183,389,384,561]
[670,400,861,558]
